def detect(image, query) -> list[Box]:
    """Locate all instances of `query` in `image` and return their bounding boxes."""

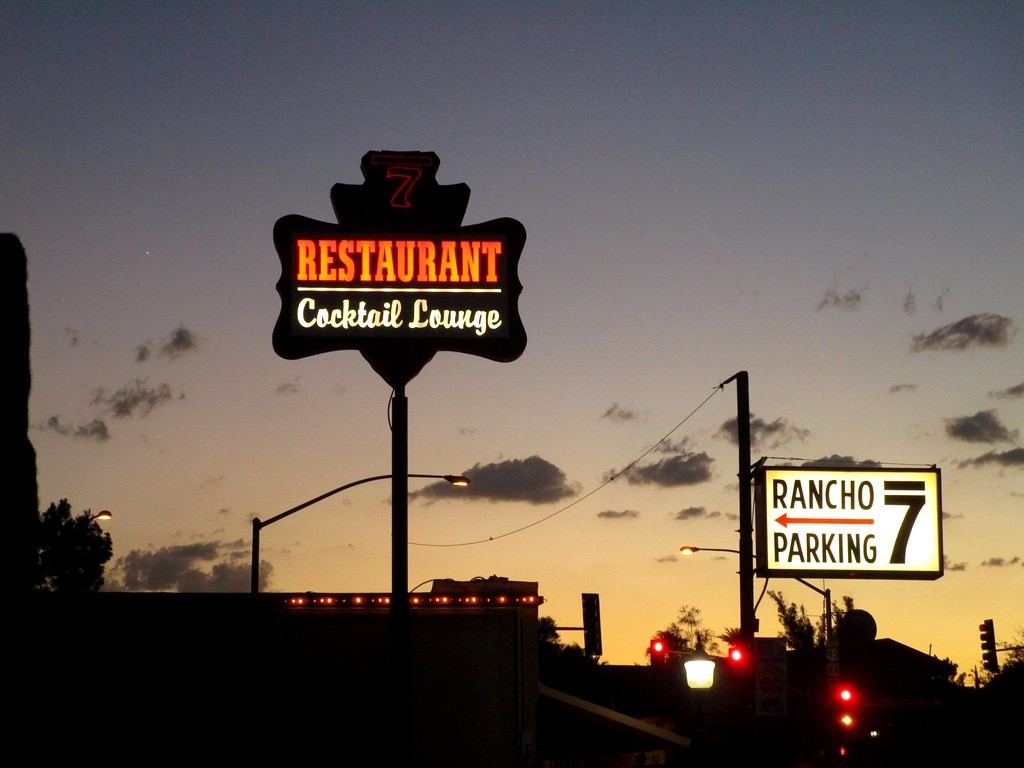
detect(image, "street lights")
[250,474,473,595]
[680,545,833,640]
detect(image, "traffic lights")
[730,644,744,670]
[832,680,864,735]
[650,638,666,668]
[980,620,1001,673]
